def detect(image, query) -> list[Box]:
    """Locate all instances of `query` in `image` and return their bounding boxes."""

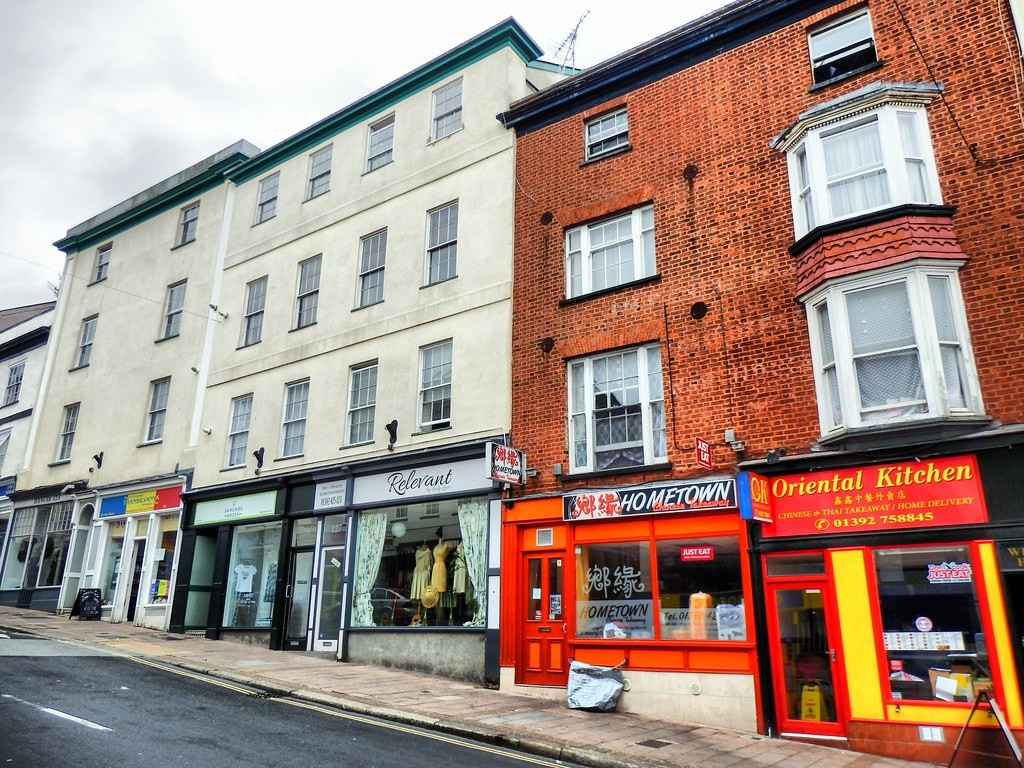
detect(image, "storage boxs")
[929,666,993,702]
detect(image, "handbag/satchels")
[567,657,626,712]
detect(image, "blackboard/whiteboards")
[945,689,1024,768]
[71,588,103,618]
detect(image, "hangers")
[236,560,256,569]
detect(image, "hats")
[421,585,440,608]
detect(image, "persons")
[264,560,279,602]
[26,538,43,587]
[409,545,432,600]
[431,538,448,592]
[452,542,471,594]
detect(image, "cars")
[369,586,434,623]
